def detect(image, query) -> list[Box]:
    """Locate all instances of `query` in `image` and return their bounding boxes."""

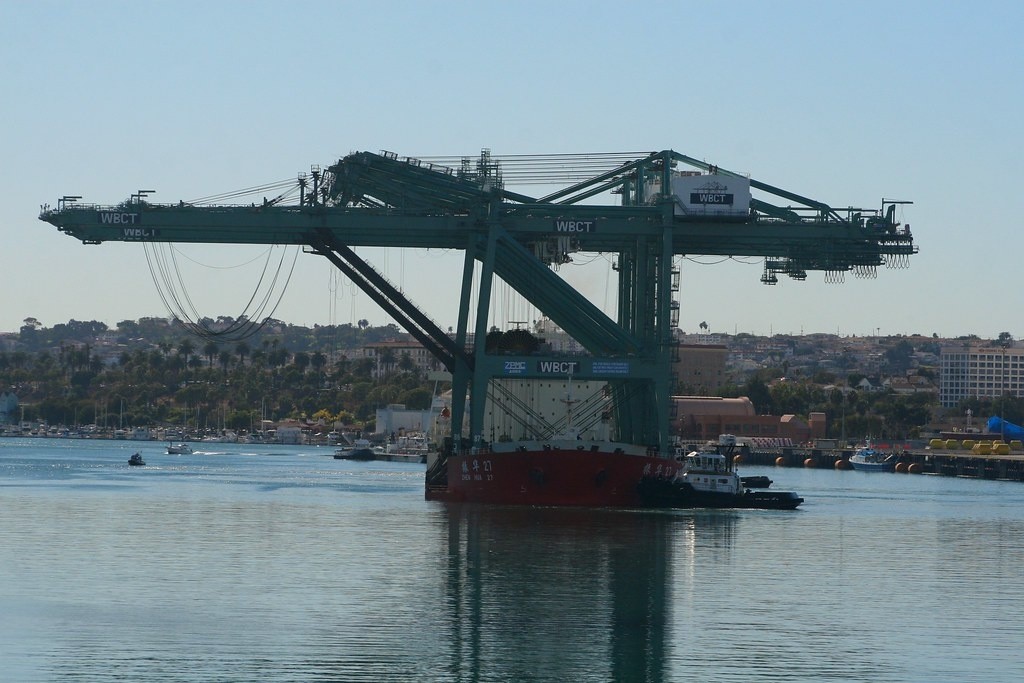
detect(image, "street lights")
[1000,342,1006,445]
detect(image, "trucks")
[718,434,737,446]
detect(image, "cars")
[22,423,32,429]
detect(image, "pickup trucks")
[316,433,327,437]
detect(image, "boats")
[848,448,899,472]
[128,452,146,465]
[165,441,193,454]
[39,150,920,511]
